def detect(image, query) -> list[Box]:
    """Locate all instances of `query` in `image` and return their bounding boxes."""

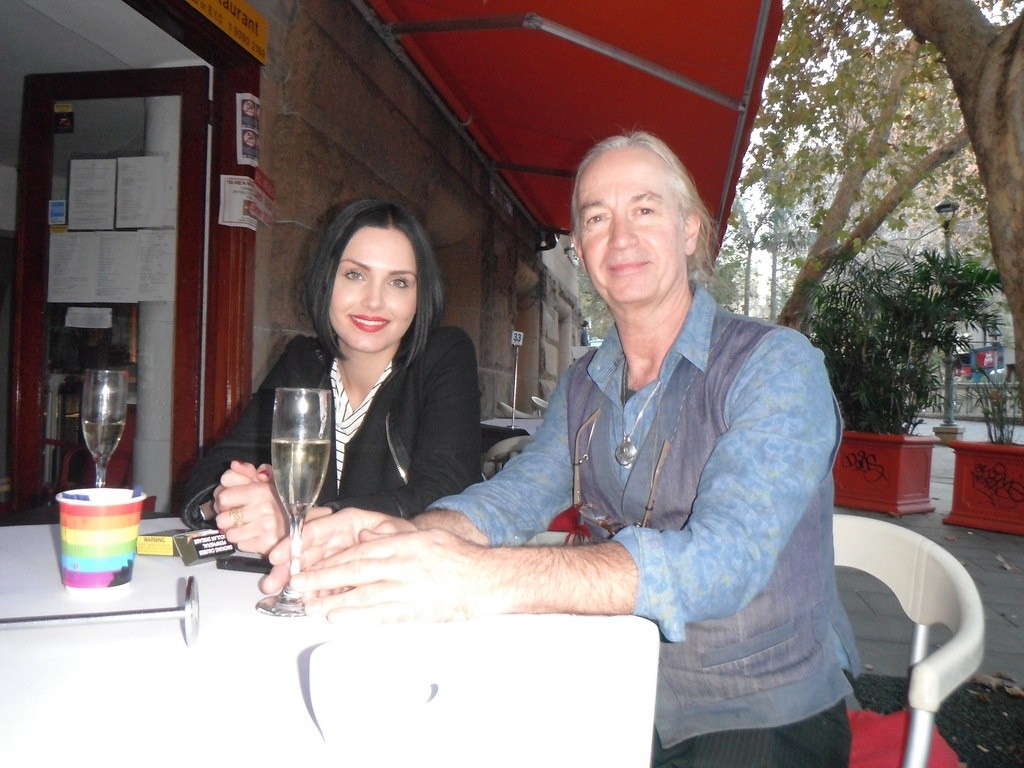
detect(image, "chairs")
[831,513,985,768]
[481,423,533,483]
[498,395,551,419]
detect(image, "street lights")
[932,193,968,441]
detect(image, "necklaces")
[614,359,661,466]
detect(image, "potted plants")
[794,243,1004,517]
[942,366,1024,536]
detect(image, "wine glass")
[255,387,333,617]
[80,369,128,489]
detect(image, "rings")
[230,508,244,528]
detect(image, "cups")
[56,488,147,589]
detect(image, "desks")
[480,417,544,437]
[1,516,660,768]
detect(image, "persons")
[258,132,864,767]
[173,197,482,560]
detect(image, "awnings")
[357,0,786,271]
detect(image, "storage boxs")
[136,528,236,567]
